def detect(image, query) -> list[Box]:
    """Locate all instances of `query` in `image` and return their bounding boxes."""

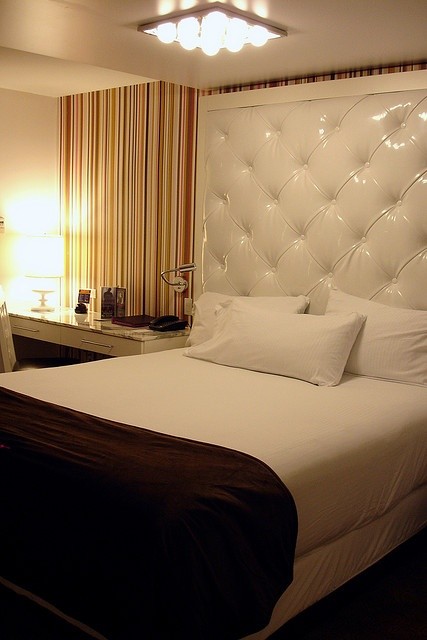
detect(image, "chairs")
[0,301,73,373]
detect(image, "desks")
[9,307,192,357]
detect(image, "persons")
[103,288,113,302]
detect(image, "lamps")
[161,263,197,293]
[21,253,64,311]
[137,1,288,56]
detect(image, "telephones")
[149,315,188,332]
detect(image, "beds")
[2,345,427,639]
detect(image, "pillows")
[324,288,427,387]
[183,300,365,388]
[185,292,311,349]
